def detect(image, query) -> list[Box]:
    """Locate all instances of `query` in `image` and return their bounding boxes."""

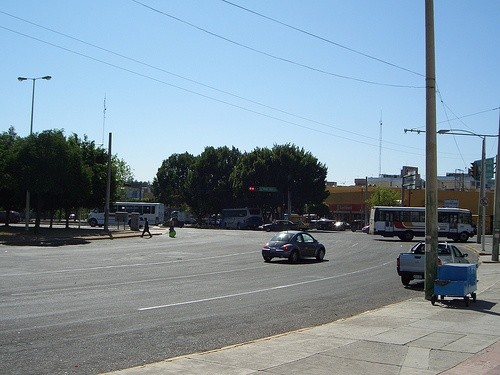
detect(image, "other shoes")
[149,236,152,238]
[141,236,143,238]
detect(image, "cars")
[362,225,369,233]
[263,232,325,265]
[0,211,21,223]
[353,220,367,230]
[69,213,75,219]
[262,220,298,232]
[334,222,351,231]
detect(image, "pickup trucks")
[396,242,470,290]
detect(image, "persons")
[141,218,153,238]
[169,217,176,237]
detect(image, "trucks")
[170,210,191,223]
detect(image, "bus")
[369,204,475,243]
[86,202,166,228]
[283,214,307,231]
[302,214,319,229]
[220,208,264,230]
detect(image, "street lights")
[439,126,487,254]
[18,75,52,234]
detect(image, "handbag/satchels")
[169,230,176,237]
